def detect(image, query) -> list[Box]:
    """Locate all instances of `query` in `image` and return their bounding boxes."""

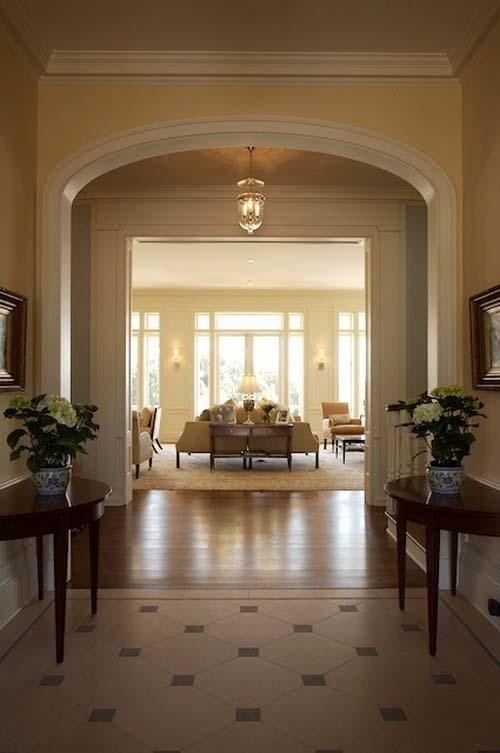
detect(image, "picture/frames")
[469,284,500,391]
[0,287,28,391]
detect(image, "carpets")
[133,443,365,491]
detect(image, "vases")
[31,464,71,495]
[426,460,466,494]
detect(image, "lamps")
[235,147,266,235]
[236,373,262,425]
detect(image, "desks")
[383,471,500,656]
[0,473,112,663]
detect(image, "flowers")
[3,394,99,473]
[398,384,487,467]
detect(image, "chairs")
[132,399,365,479]
